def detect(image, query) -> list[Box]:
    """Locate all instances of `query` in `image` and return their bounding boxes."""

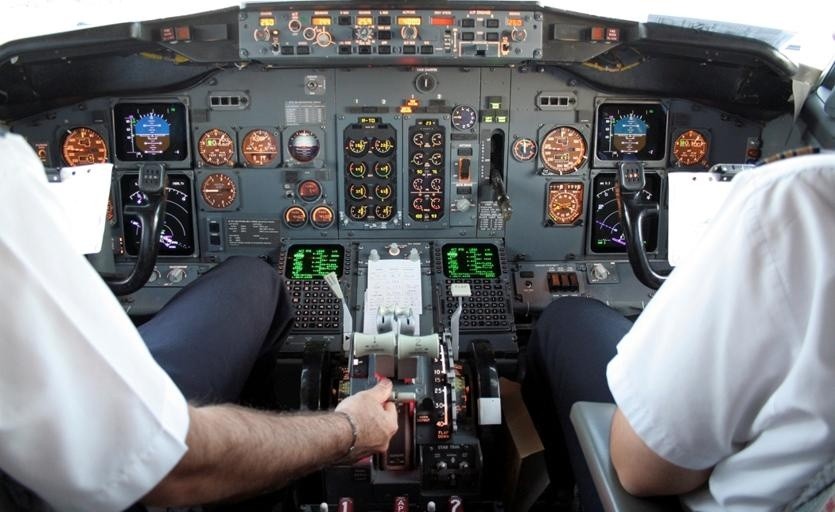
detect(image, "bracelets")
[337,410,357,462]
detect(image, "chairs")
[570,402,834,511]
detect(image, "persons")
[524,143,833,512]
[0,128,399,511]
